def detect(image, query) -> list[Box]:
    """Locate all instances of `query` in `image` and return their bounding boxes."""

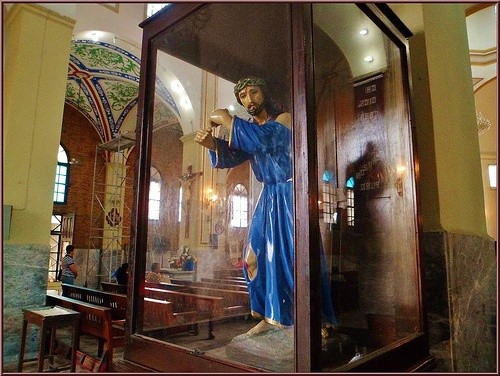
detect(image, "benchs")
[46,277,250,373]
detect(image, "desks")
[160,268,195,281]
[19,304,81,373]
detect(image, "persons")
[194,72,336,338]
[61,245,80,296]
[114,262,128,285]
[145,262,161,284]
